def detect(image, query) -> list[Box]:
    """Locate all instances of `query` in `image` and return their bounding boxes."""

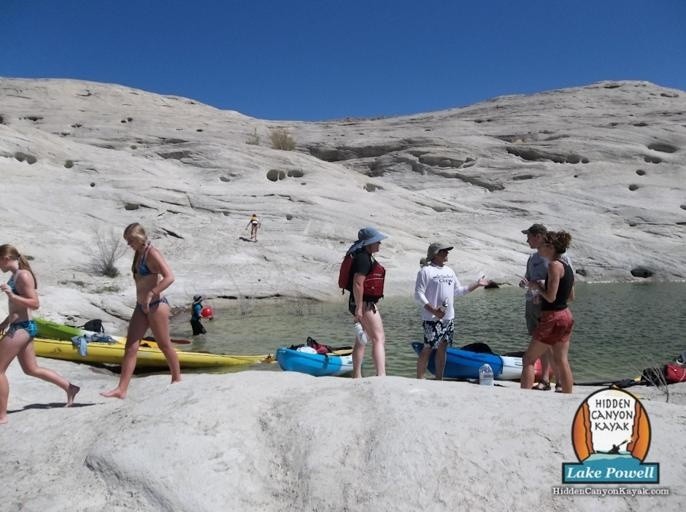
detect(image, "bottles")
[352,321,368,348]
[436,304,448,321]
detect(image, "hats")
[424,242,454,261]
[192,294,206,305]
[345,227,388,255]
[522,223,546,235]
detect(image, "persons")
[347,227,389,379]
[245,213,262,242]
[189,294,214,336]
[1,242,80,427]
[415,241,492,382]
[100,223,181,399]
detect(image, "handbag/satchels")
[306,336,330,353]
[661,362,686,383]
[86,318,103,331]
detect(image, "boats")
[412,341,552,378]
[0,316,353,376]
